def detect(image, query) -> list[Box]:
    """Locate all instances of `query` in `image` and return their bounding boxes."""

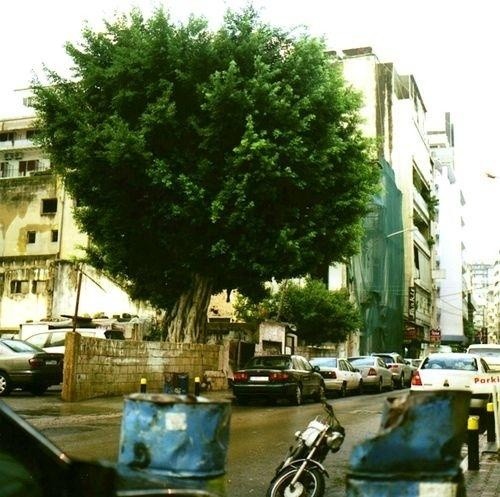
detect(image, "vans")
[25,327,126,396]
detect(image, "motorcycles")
[266,396,348,497]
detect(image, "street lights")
[383,227,419,309]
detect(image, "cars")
[0,336,64,399]
[0,397,221,497]
[230,340,500,410]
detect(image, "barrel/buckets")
[116,393,232,496]
